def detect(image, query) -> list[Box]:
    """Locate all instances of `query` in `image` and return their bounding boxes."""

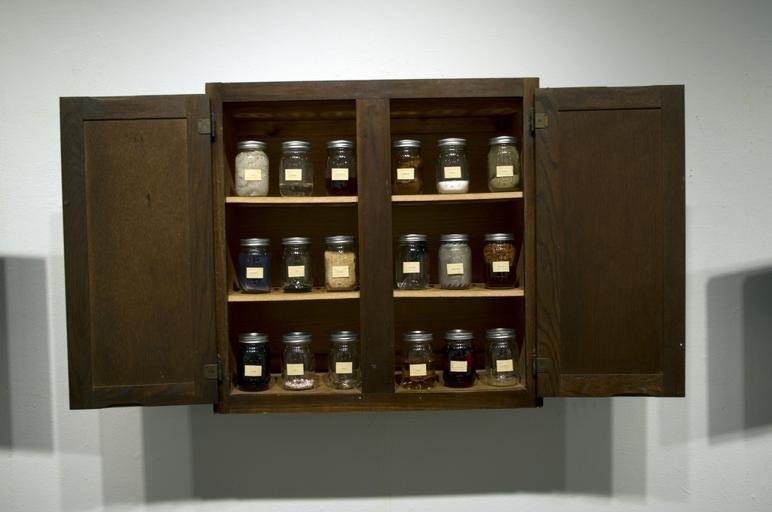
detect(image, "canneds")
[326,330,362,389]
[235,331,272,391]
[487,135,521,192]
[435,137,471,194]
[482,232,518,289]
[325,139,358,196]
[278,140,314,196]
[234,140,270,197]
[323,235,358,292]
[237,236,272,294]
[391,139,426,195]
[440,328,476,388]
[484,326,522,387]
[436,234,472,289]
[279,332,319,390]
[395,233,430,290]
[400,329,438,389]
[279,236,314,292]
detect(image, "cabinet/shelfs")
[57,76,687,416]
